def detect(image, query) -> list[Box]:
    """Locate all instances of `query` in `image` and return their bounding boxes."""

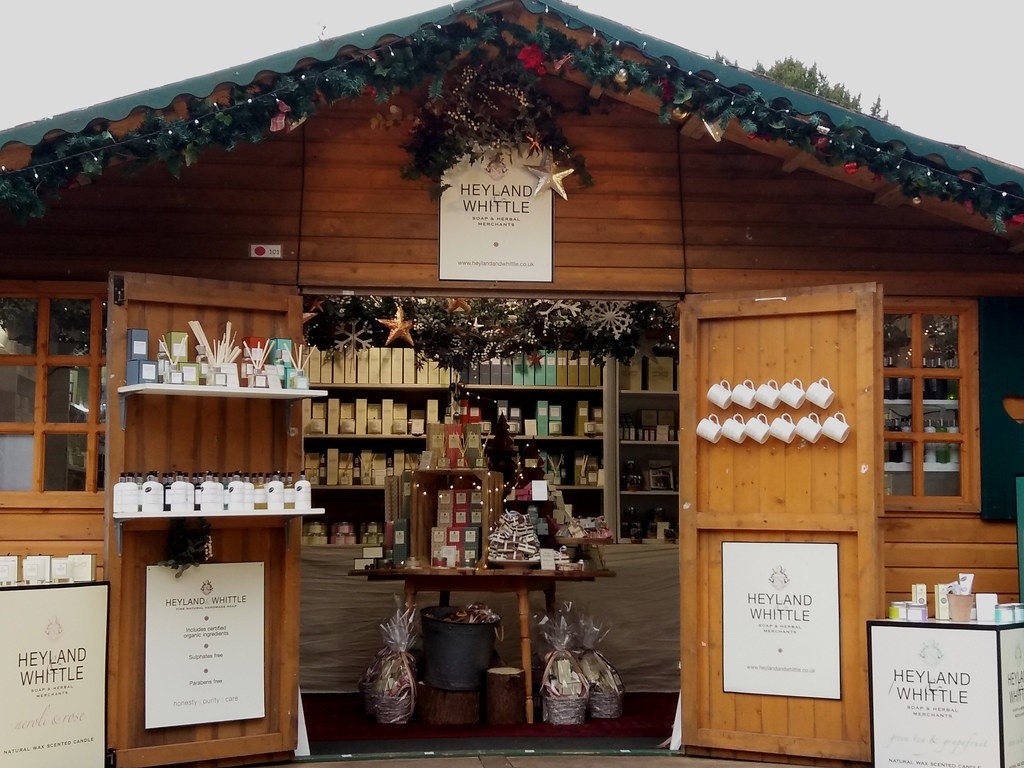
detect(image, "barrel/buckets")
[420,605,502,691]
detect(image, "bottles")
[241,348,254,387]
[883,321,960,465]
[576,545,599,570]
[290,370,309,390]
[301,521,384,544]
[971,601,1024,623]
[195,345,209,385]
[163,364,184,384]
[274,349,287,389]
[248,369,269,388]
[620,461,643,491]
[889,600,928,622]
[206,367,228,386]
[157,342,169,383]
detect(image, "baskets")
[578,654,623,719]
[359,653,416,726]
[541,654,590,726]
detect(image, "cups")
[795,413,822,444]
[822,412,850,444]
[707,380,732,410]
[696,414,722,444]
[769,413,796,444]
[806,378,835,410]
[721,413,747,444]
[745,413,770,444]
[778,379,806,409]
[730,379,757,410]
[754,380,782,410]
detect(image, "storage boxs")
[0,554,96,583]
[910,583,998,622]
[125,328,674,571]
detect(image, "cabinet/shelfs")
[112,383,328,557]
[300,354,680,694]
[883,398,962,472]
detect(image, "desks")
[347,566,617,724]
